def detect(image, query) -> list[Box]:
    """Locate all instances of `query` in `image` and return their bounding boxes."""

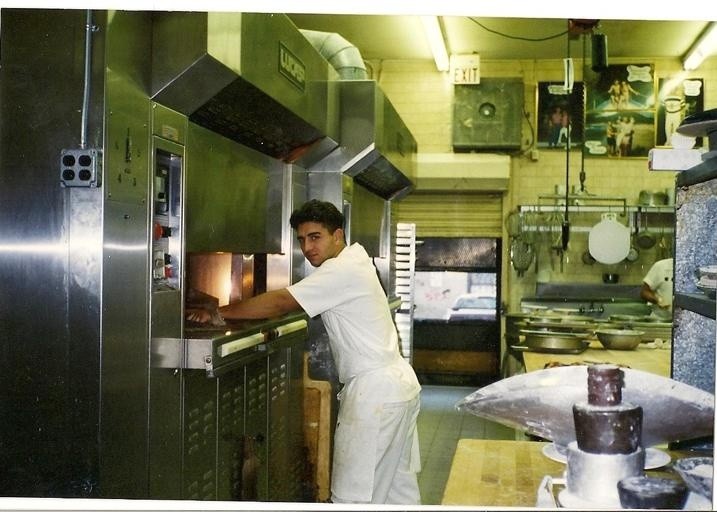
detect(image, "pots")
[636,212,657,249]
[626,246,640,263]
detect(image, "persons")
[186,200,423,501]
[640,257,676,321]
[547,78,687,157]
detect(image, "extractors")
[296,29,418,203]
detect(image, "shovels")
[658,218,665,248]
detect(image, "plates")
[542,442,672,471]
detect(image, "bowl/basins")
[502,312,654,333]
[674,457,714,499]
[518,330,590,350]
[595,330,646,351]
[629,322,673,343]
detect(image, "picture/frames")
[534,61,706,161]
